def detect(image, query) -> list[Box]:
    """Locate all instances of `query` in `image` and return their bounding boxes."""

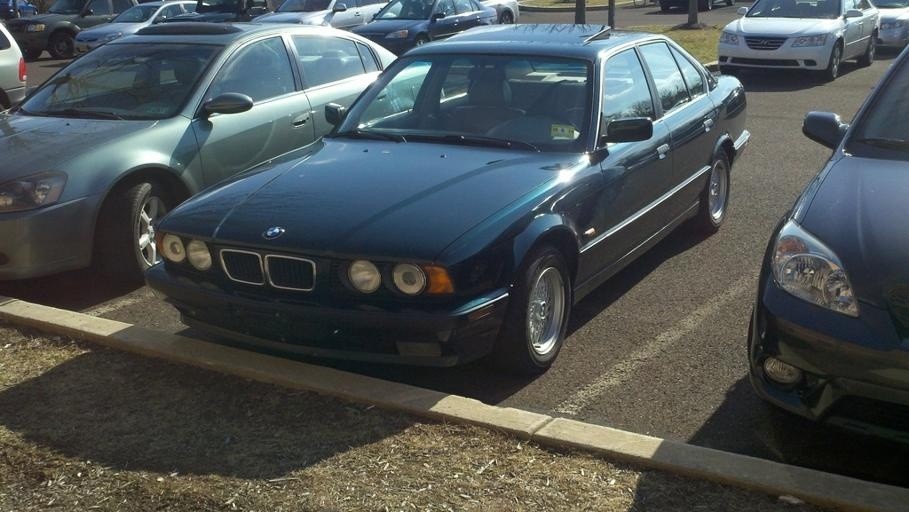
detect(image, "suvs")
[5,1,145,58]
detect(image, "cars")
[659,0,735,11]
[852,0,909,52]
[250,1,403,30]
[0,20,446,285]
[717,0,880,81]
[482,0,518,24]
[350,1,497,55]
[167,1,280,29]
[0,22,29,115]
[144,24,751,376]
[747,37,909,447]
[75,1,209,55]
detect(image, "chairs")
[522,76,588,142]
[445,70,523,137]
[242,49,282,99]
[168,56,201,99]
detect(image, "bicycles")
[634,1,660,8]
